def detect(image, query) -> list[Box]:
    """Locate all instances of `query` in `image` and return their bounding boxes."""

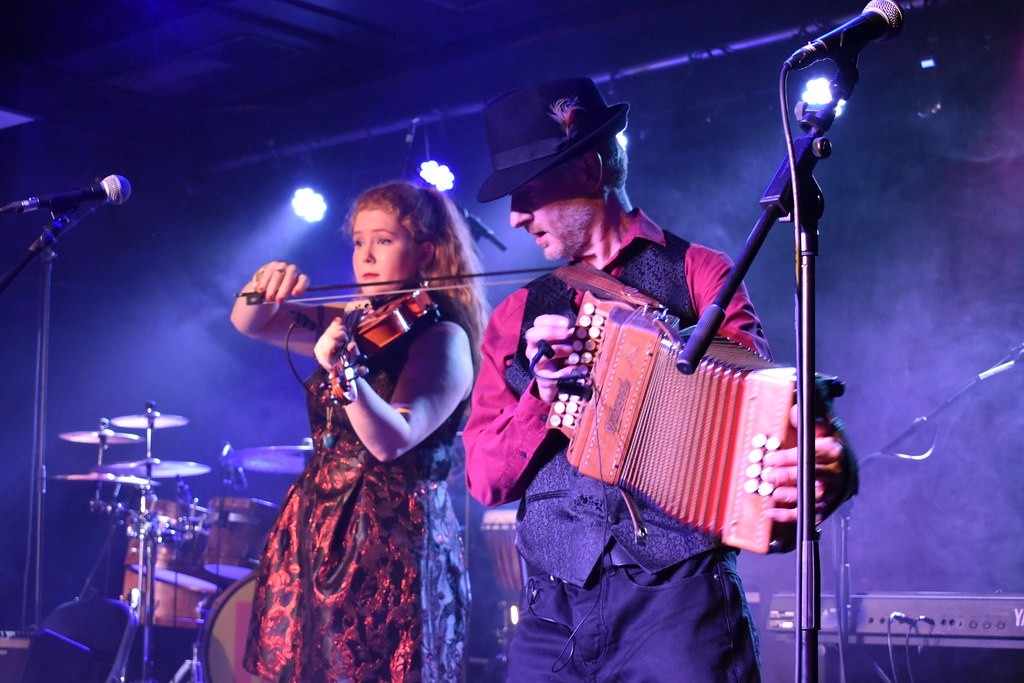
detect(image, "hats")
[477,77,629,203]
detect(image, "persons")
[231,181,491,683]
[462,77,859,683]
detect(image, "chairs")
[791,37,847,118]
[201,532,267,590]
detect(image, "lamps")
[290,155,329,223]
[413,135,455,192]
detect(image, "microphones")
[225,442,248,491]
[292,313,315,331]
[532,339,555,363]
[0,174,132,215]
[786,0,905,72]
[175,476,190,504]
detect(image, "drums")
[205,497,283,580]
[196,569,268,682]
[121,495,224,630]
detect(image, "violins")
[317,287,434,410]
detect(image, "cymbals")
[90,459,214,479]
[54,472,163,489]
[57,430,146,446]
[218,444,314,474]
[111,413,190,429]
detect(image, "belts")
[525,542,642,576]
